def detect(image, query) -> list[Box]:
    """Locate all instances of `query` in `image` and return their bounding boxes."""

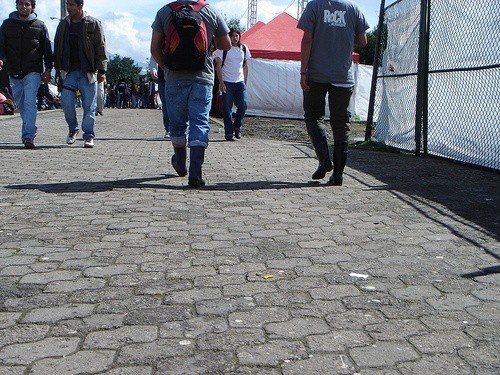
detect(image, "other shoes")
[330,176,343,185]
[312,158,333,179]
[226,136,234,141]
[234,130,241,139]
[23,138,36,150]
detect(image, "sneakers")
[84,137,95,147]
[66,130,79,144]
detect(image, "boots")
[170,143,188,177]
[188,146,207,187]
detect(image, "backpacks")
[160,1,209,71]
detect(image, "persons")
[54,0,106,148]
[213,28,252,140]
[296,0,369,185]
[0,0,53,148]
[149,0,232,186]
[104,76,163,110]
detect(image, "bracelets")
[300,72,308,75]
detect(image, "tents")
[212,12,359,121]
